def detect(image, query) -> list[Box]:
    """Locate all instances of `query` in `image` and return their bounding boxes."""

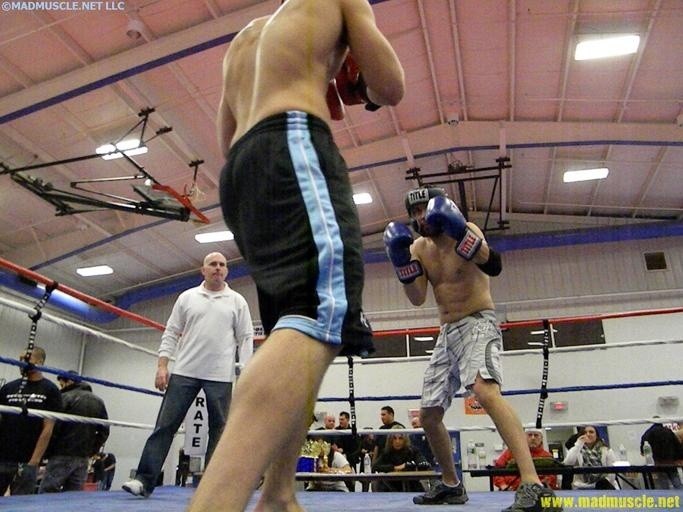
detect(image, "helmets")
[405,186,448,237]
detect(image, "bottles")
[619,443,628,462]
[362,453,371,475]
[477,446,486,473]
[466,440,477,470]
[642,441,654,467]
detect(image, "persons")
[638,414,681,489]
[96,452,115,490]
[37,370,109,492]
[0,347,62,496]
[382,182,562,509]
[491,422,556,491]
[121,251,256,499]
[185,0,408,512]
[558,423,622,490]
[311,405,431,491]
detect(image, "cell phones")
[580,429,585,435]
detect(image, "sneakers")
[413,481,469,505]
[501,481,563,511]
[121,478,150,498]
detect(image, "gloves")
[326,48,384,120]
[425,196,482,262]
[383,221,424,284]
[9,464,37,494]
